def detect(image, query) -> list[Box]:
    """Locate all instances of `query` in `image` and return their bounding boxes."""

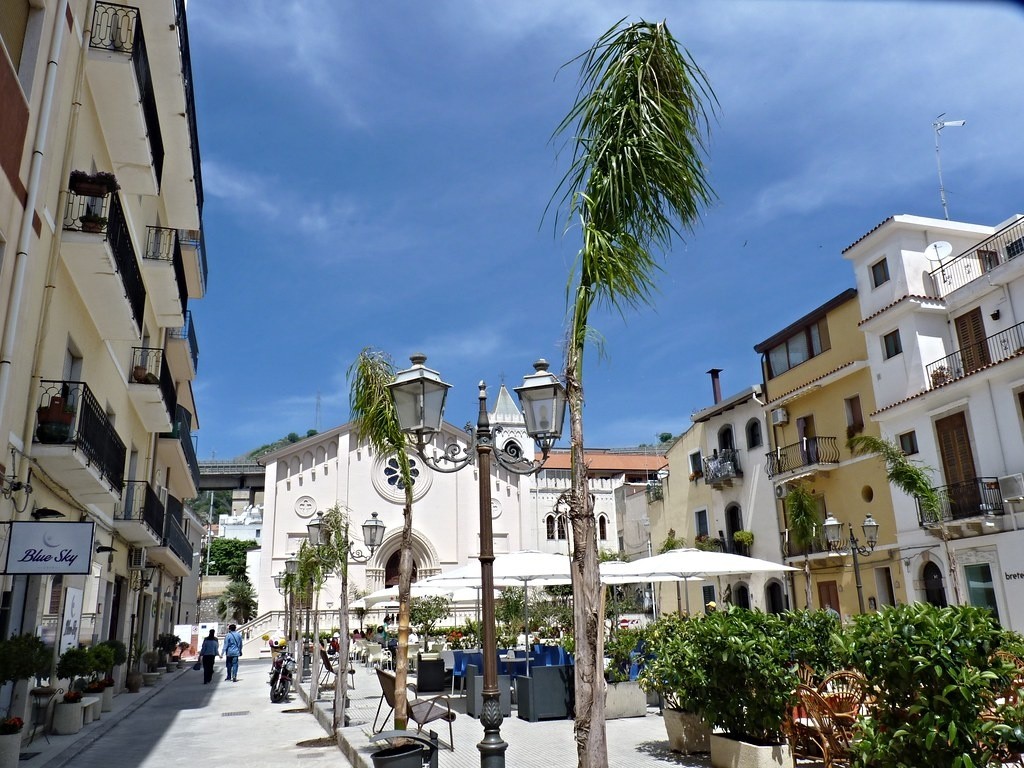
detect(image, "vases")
[36,421,70,445]
[134,365,158,384]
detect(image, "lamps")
[31,508,66,520]
[96,546,118,554]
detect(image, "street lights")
[381,352,569,768]
[271,553,333,677]
[305,510,387,708]
[823,512,880,615]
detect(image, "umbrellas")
[350,549,803,677]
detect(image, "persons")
[381,616,392,639]
[373,626,386,647]
[706,601,717,611]
[198,629,223,684]
[353,629,361,638]
[332,632,340,644]
[408,627,418,642]
[682,609,690,621]
[221,624,242,682]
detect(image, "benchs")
[372,665,456,752]
[317,649,355,691]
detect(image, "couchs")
[467,664,576,722]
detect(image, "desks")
[497,656,535,676]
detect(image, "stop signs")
[620,619,628,629]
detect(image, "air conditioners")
[771,408,788,426]
[774,482,788,499]
[130,546,147,569]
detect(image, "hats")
[706,600,716,608]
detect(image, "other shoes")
[233,676,236,682]
[225,679,231,681]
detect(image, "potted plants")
[632,608,733,755]
[68,169,121,233]
[733,530,754,557]
[686,604,847,768]
[847,602,1024,768]
[37,396,77,426]
[0,632,190,768]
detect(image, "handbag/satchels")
[193,661,201,671]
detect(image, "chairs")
[781,650,1024,768]
[348,638,446,671]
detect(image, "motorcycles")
[266,649,297,703]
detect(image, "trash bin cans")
[368,729,438,768]
